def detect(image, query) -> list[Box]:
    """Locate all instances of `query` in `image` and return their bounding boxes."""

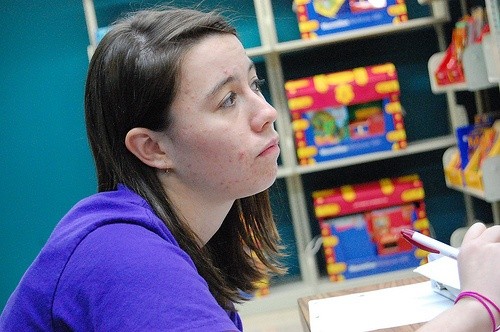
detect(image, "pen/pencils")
[398,226,459,262]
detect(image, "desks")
[297,274,442,332]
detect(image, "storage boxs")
[465,117,498,189]
[285,62,409,167]
[291,0,408,40]
[436,17,490,87]
[445,127,489,187]
[312,173,435,281]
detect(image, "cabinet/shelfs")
[82,0,500,332]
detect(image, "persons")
[0,9,500,332]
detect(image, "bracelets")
[451,288,500,331]
